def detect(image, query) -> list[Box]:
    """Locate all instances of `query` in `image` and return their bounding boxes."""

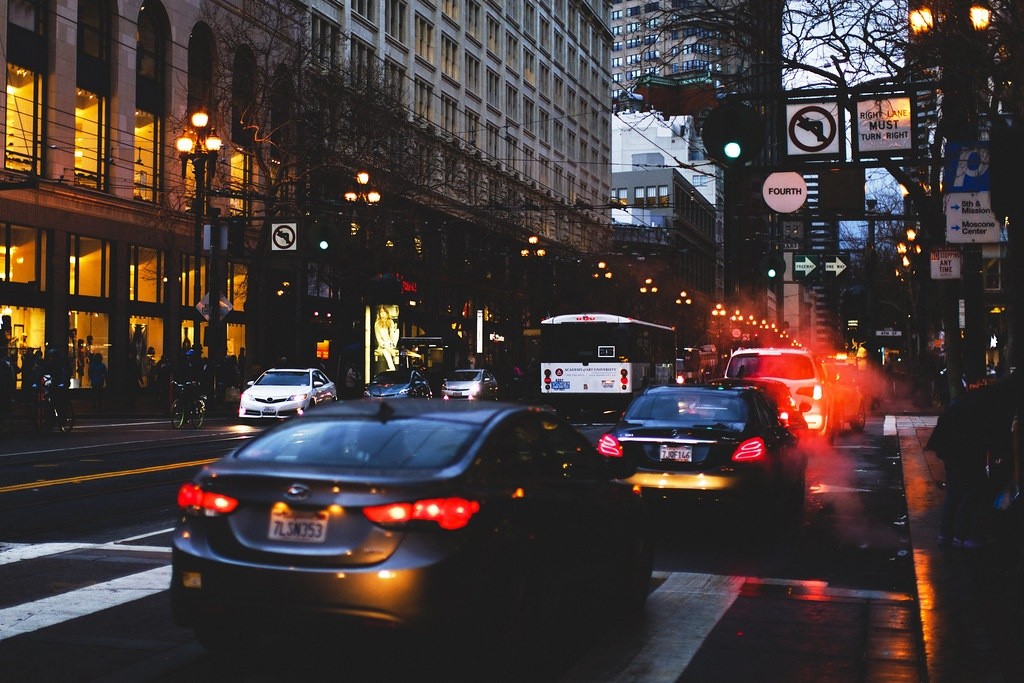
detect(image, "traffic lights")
[699,104,767,172]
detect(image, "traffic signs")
[823,254,851,279]
[793,254,819,278]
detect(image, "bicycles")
[170,380,208,430]
[30,372,75,436]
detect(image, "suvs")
[236,366,338,428]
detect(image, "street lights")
[592,259,612,315]
[343,168,382,401]
[712,303,726,378]
[746,314,757,349]
[675,289,692,359]
[520,235,546,346]
[174,109,223,414]
[732,309,744,351]
[903,0,1010,559]
[639,277,658,322]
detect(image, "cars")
[595,381,799,539]
[363,368,433,400]
[165,395,656,683]
[441,369,500,403]
[699,378,814,488]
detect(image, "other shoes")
[951,537,978,548]
[937,535,951,545]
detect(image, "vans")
[721,346,869,452]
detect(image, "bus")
[536,312,678,423]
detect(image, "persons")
[88,353,108,411]
[926,369,1024,548]
[0,347,42,414]
[76,335,94,386]
[346,361,362,395]
[8,333,28,367]
